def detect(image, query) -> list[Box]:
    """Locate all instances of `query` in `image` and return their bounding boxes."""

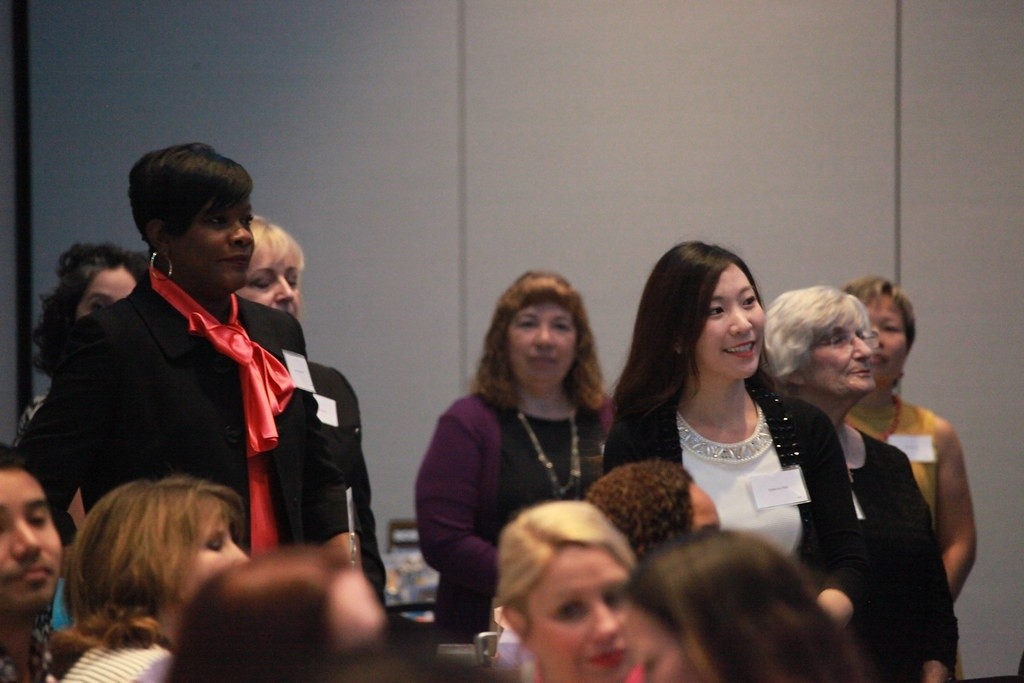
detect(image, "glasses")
[813,331,881,351]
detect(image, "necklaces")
[843,391,903,442]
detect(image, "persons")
[764,287,960,682]
[516,399,583,493]
[491,500,644,683]
[16,239,148,446]
[586,456,721,559]
[0,444,69,683]
[55,473,249,683]
[835,274,978,604]
[618,526,875,683]
[601,240,873,629]
[414,267,618,642]
[14,142,316,555]
[230,214,389,609]
[166,543,389,682]
